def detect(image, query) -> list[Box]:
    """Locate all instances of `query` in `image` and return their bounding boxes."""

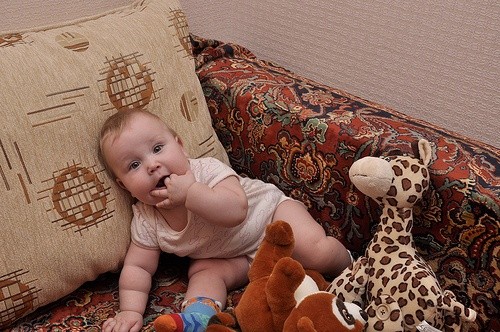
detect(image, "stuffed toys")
[325,139,477,332]
[207,220,368,332]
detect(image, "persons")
[97,108,355,332]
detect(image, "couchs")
[0,32,500,332]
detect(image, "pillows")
[0,0,236,332]
